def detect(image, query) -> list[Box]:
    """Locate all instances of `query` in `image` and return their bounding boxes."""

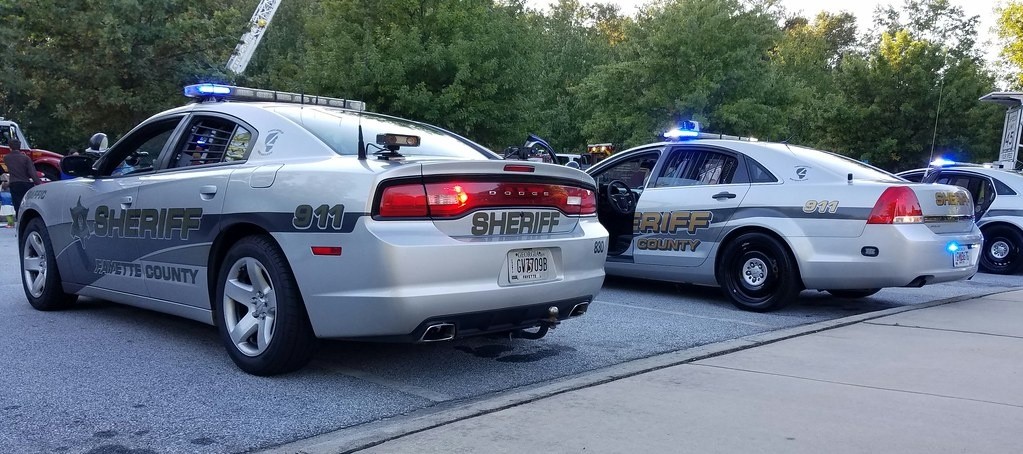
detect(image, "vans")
[542,153,597,171]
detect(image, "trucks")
[0,117,65,183]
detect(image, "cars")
[520,120,985,314]
[17,84,612,376]
[895,159,1023,276]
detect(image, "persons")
[0,182,16,227]
[61,150,80,180]
[3,138,40,221]
